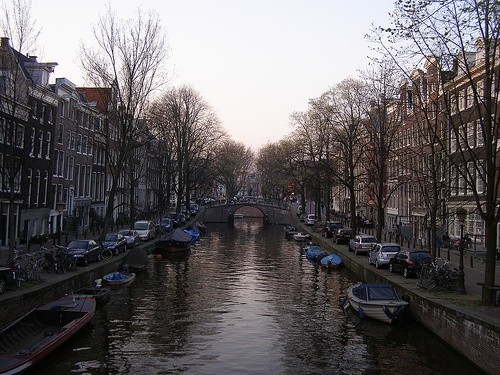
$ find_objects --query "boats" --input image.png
[284,225,344,269]
[102,247,161,285]
[234,212,243,219]
[0,282,112,375]
[342,282,409,324]
[160,221,208,252]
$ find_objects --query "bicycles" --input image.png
[98,242,113,260]
[418,257,460,290]
[6,243,76,288]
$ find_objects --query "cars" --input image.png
[332,228,353,244]
[63,239,101,266]
[102,229,140,256]
[155,204,199,232]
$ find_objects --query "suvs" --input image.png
[304,214,318,225]
[368,242,432,278]
[320,221,344,238]
[349,234,378,255]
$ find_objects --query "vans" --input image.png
[134,220,157,241]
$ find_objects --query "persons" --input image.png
[394,225,400,243]
[356,214,362,226]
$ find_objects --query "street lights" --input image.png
[456,206,467,293]
[357,204,361,235]
